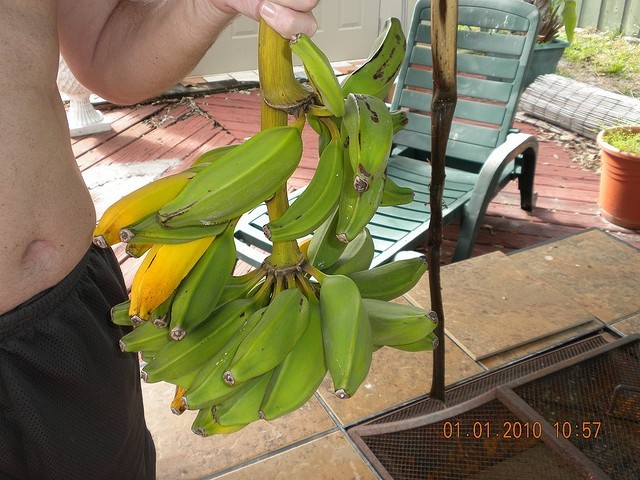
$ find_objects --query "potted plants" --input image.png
[486,0,577,92]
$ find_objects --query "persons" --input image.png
[1,0,321,479]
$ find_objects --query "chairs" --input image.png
[232,0,541,287]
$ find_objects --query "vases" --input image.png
[597,124,640,230]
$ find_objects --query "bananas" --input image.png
[87,17,415,343]
[90,228,440,439]
